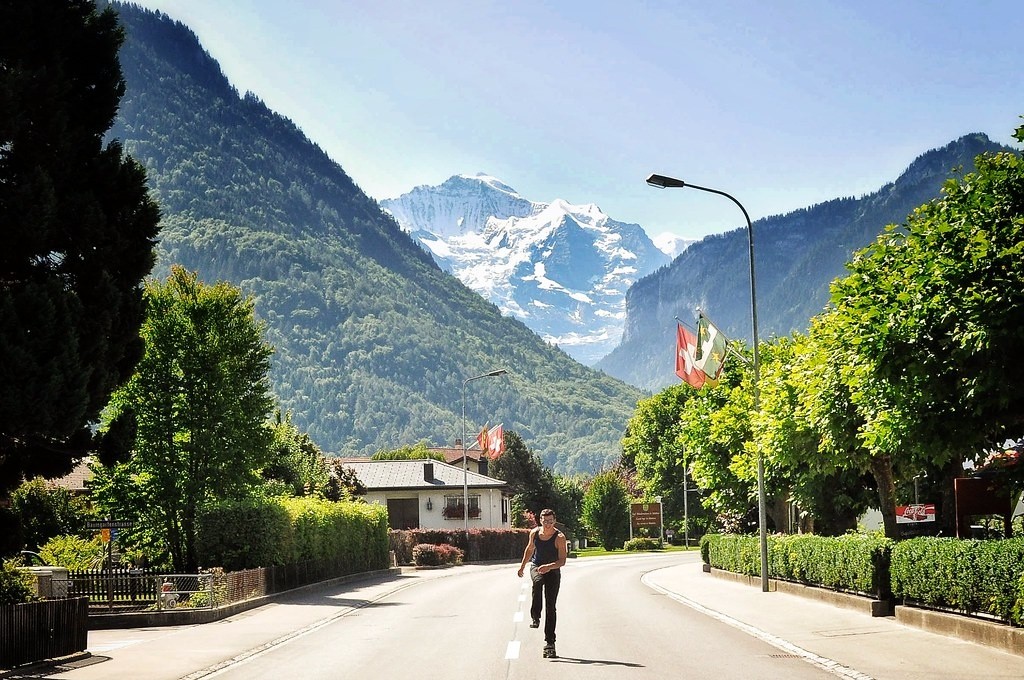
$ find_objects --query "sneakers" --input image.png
[529,617,541,628]
[543,643,555,652]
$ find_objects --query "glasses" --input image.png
[541,519,556,525]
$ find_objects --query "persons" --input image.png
[518,509,566,649]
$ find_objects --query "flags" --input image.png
[674,321,706,389]
[694,311,734,380]
[487,426,504,459]
[477,425,490,451]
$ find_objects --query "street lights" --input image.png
[646,174,769,592]
[955,478,980,538]
[464,369,505,546]
